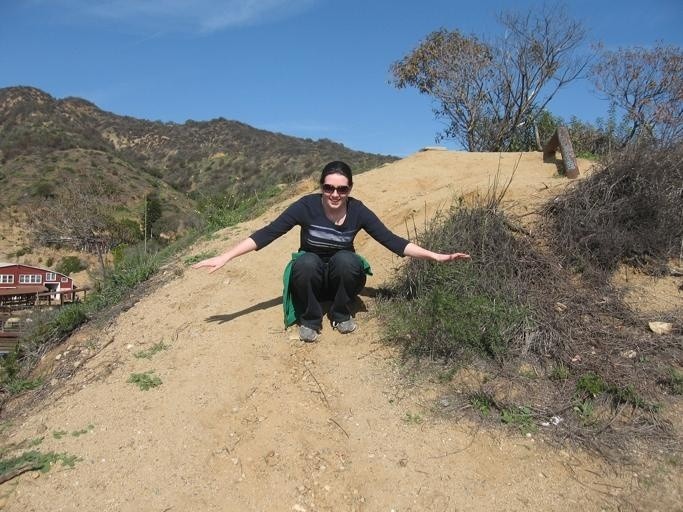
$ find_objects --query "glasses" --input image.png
[320,183,351,195]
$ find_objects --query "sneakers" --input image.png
[299,325,318,342]
[333,313,356,334]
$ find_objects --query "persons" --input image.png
[191,161,470,341]
